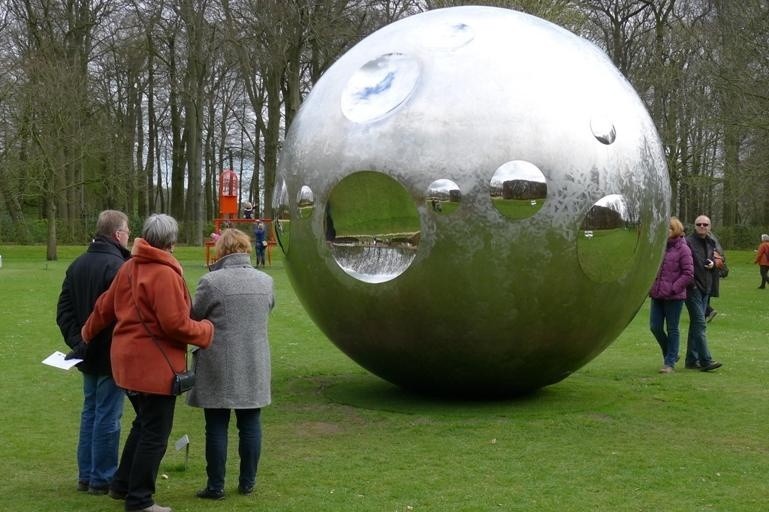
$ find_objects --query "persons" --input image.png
[648,215,695,374]
[253,219,266,269]
[685,215,723,372]
[56,208,134,496]
[208,232,222,242]
[80,212,215,512]
[189,228,275,498]
[753,234,769,289]
[240,201,259,219]
[705,230,729,324]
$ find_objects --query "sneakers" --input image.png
[705,309,717,324]
[758,286,765,290]
[127,504,172,512]
[76,478,89,491]
[685,362,701,369]
[88,485,107,495]
[238,487,254,495]
[196,488,225,499]
[109,488,127,499]
[701,360,722,372]
[673,355,680,363]
[659,366,674,374]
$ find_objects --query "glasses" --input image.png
[119,229,132,236]
[696,223,710,227]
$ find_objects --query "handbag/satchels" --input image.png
[172,371,196,396]
[261,240,268,248]
